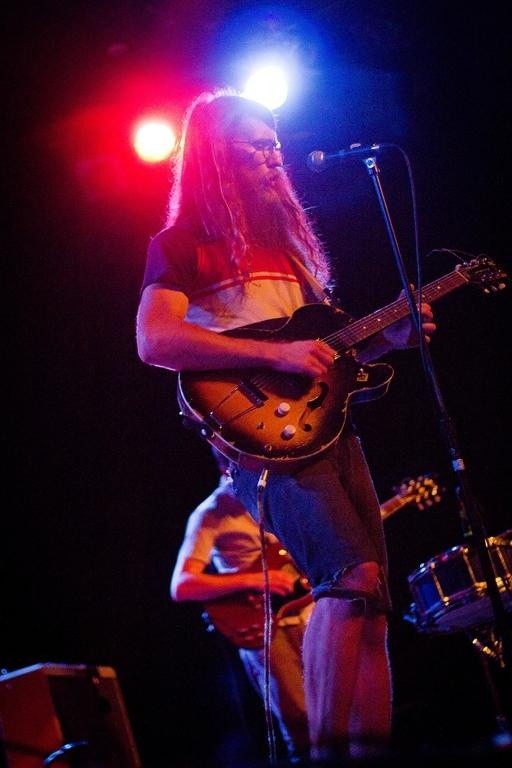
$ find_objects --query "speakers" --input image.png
[5,652,136,759]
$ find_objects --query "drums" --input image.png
[407,531,512,633]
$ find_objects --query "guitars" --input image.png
[200,476,442,650]
[175,255,508,476]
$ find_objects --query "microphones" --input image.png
[305,141,393,174]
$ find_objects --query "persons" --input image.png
[171,445,314,768]
[135,94,435,767]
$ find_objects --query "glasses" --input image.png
[231,138,284,152]
